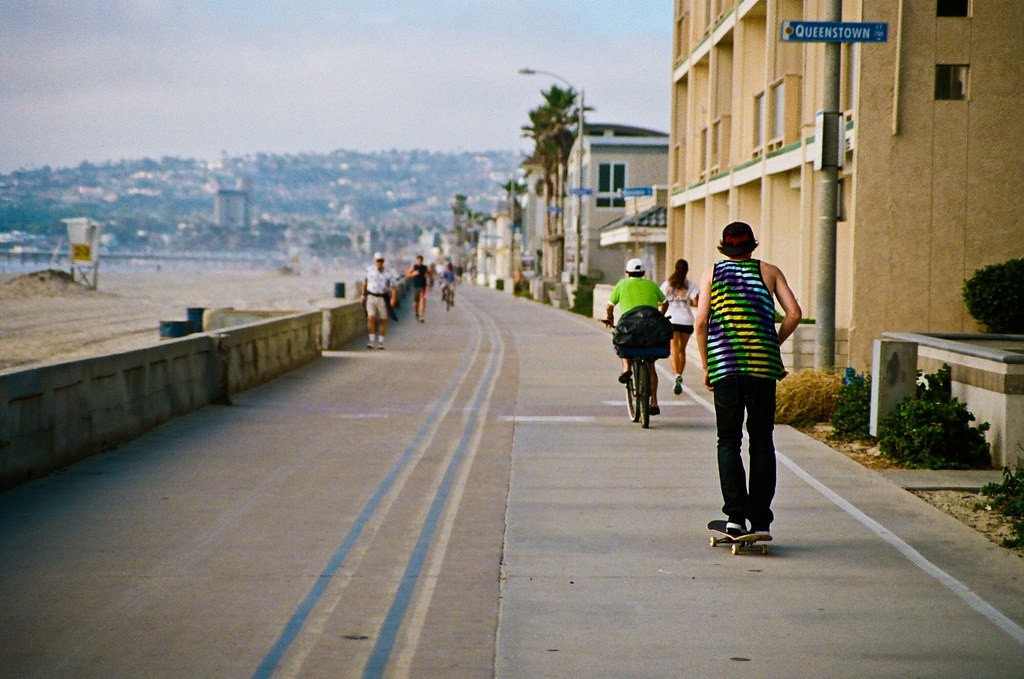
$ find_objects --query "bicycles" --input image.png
[442,278,457,311]
[601,316,672,428]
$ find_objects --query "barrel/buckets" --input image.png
[158,319,196,344]
[188,307,211,333]
[334,281,346,299]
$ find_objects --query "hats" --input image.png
[374,252,384,261]
[626,258,644,272]
[719,222,756,256]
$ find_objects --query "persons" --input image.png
[513,267,524,298]
[694,221,803,536]
[605,258,669,415]
[660,259,700,396]
[404,255,478,322]
[360,253,398,350]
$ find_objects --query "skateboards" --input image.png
[705,519,774,556]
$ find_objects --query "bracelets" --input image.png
[361,293,366,297]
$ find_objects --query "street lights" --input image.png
[518,67,584,293]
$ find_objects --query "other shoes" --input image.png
[442,294,445,300]
[749,525,768,533]
[378,341,384,348]
[650,405,660,415]
[418,317,424,321]
[367,341,374,347]
[726,516,746,534]
[619,369,632,383]
[673,373,682,394]
